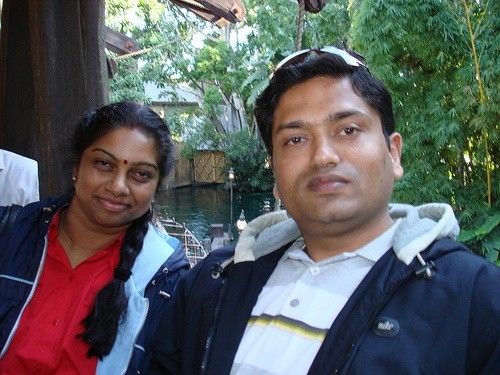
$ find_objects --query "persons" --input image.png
[145,49,500,374]
[0,102,191,375]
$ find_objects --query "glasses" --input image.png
[269,44,372,81]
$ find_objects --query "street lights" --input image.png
[228,167,235,224]
